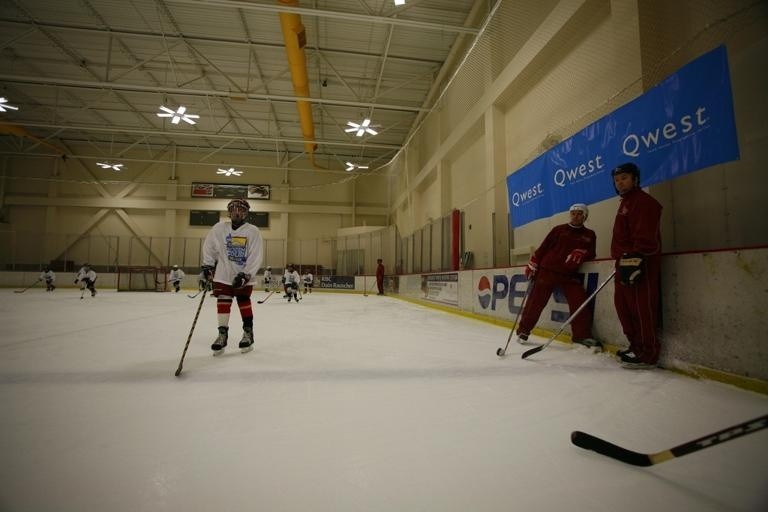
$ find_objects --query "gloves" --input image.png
[565,249,587,264]
[232,272,251,289]
[525,256,539,280]
[198,265,213,291]
[619,251,646,285]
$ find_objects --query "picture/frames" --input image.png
[191,182,270,200]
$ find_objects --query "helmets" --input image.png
[287,264,295,268]
[228,198,250,219]
[569,203,588,222]
[611,162,640,194]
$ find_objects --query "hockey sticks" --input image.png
[186,288,205,298]
[157,278,181,284]
[175,260,218,376]
[497,246,537,355]
[14,280,41,293]
[571,414,768,468]
[522,270,616,359]
[257,281,283,303]
[364,280,376,297]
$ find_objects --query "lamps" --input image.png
[345,156,368,171]
[96,160,124,172]
[0,96,19,113]
[216,167,243,177]
[344,108,378,137]
[156,104,200,125]
[394,0,405,5]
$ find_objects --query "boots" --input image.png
[239,326,253,348]
[211,326,229,350]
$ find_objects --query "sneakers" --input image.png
[516,329,528,340]
[295,297,299,302]
[287,297,291,301]
[617,348,641,363]
[572,336,602,346]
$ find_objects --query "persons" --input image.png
[73,263,97,299]
[199,268,208,291]
[518,203,602,350]
[375,258,384,295]
[264,264,313,301]
[202,197,264,350]
[168,264,185,292]
[610,161,663,365]
[38,266,56,291]
